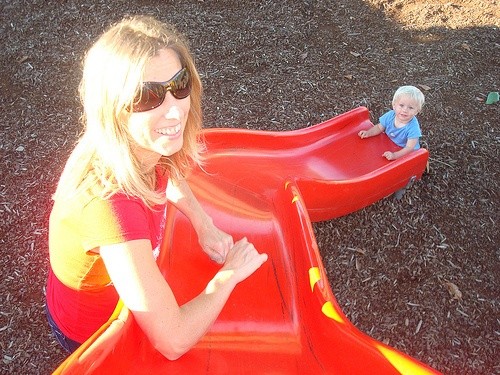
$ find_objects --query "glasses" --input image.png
[125,67,192,113]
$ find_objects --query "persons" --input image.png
[358,85,425,200]
[45,15,269,361]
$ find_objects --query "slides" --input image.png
[51,103,443,375]
